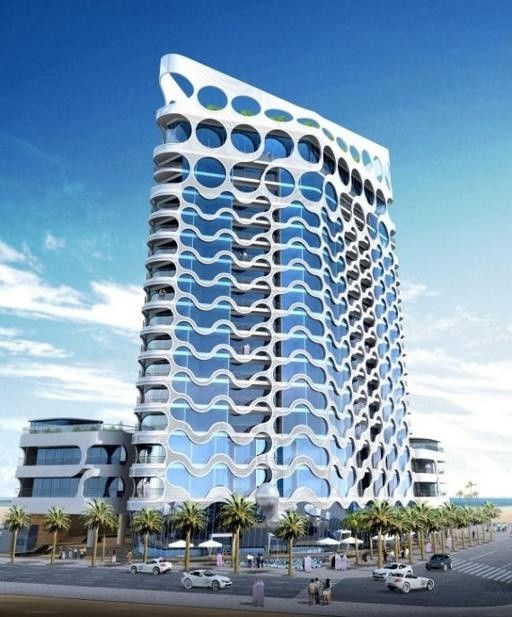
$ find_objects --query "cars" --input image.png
[425,554,453,572]
[373,563,414,581]
[129,558,173,576]
[180,569,233,591]
[385,572,434,594]
